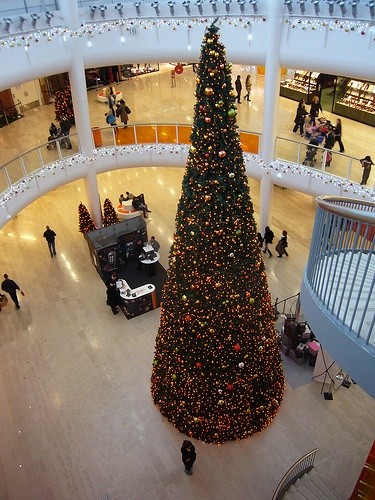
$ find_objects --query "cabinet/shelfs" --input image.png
[331,76,375,127]
[280,70,322,104]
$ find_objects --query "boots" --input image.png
[66,143,72,149]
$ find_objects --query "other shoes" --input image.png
[268,255,272,258]
[112,307,119,315]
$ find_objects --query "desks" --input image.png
[116,279,156,315]
[139,251,160,276]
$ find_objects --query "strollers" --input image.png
[46,128,67,150]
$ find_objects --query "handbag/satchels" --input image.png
[125,106,130,114]
[20,291,24,296]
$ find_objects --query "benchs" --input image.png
[116,202,143,220]
[97,91,122,103]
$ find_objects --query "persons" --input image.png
[360,156,373,185]
[150,236,160,252]
[234,76,241,104]
[119,191,152,218]
[49,83,128,150]
[257,226,289,258]
[244,75,252,101]
[181,440,196,475]
[43,226,56,259]
[292,96,345,166]
[105,273,121,315]
[0,274,23,311]
